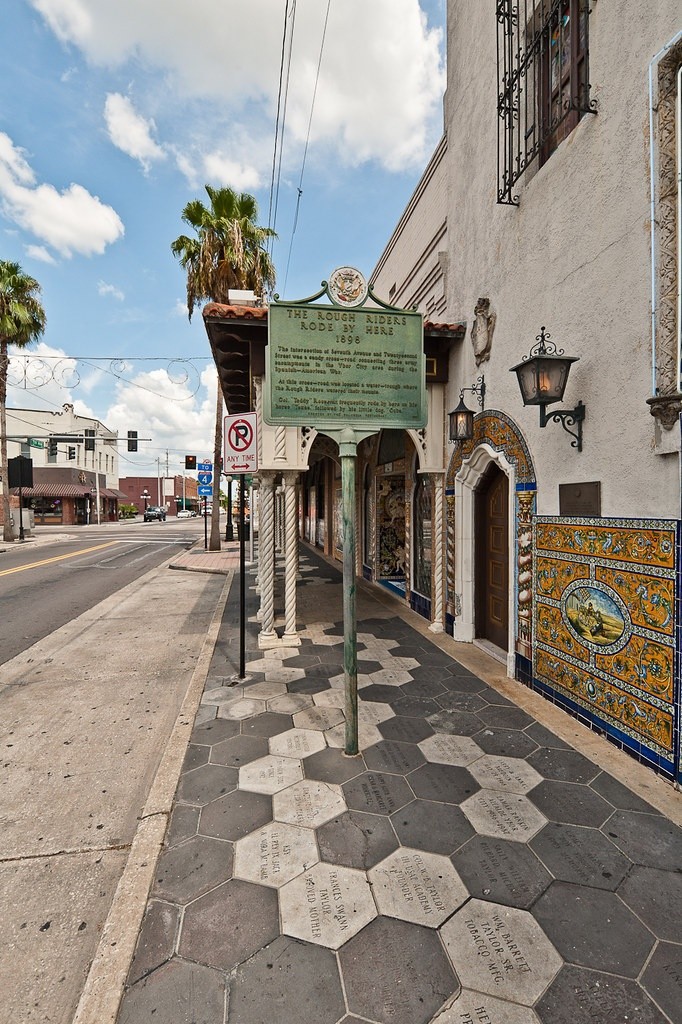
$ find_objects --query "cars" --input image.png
[191,511,196,517]
[177,509,192,518]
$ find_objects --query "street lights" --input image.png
[224,476,234,541]
[140,489,151,522]
[173,495,181,517]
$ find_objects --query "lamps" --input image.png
[508,326,585,452]
[448,375,486,451]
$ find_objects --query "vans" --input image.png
[200,505,213,517]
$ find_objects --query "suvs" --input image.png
[144,506,167,522]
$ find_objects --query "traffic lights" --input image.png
[220,458,223,470]
[49,441,57,456]
[185,455,197,470]
[68,446,76,460]
